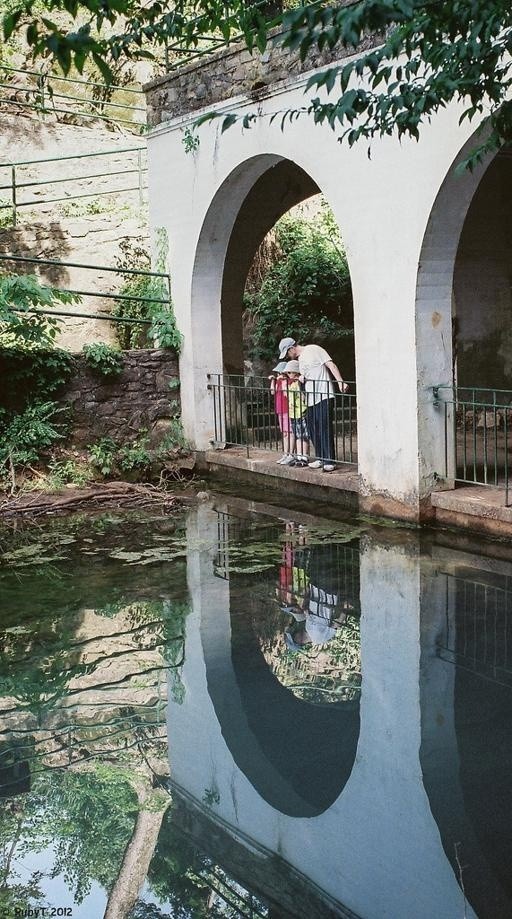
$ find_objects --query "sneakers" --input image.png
[295,459,310,466]
[288,458,299,466]
[323,464,337,471]
[308,459,323,469]
[276,455,289,463]
[280,457,294,465]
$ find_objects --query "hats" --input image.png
[278,337,296,361]
[272,362,287,373]
[282,360,300,374]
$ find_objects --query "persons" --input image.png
[279,337,350,472]
[278,360,310,466]
[268,362,295,465]
[275,522,354,651]
